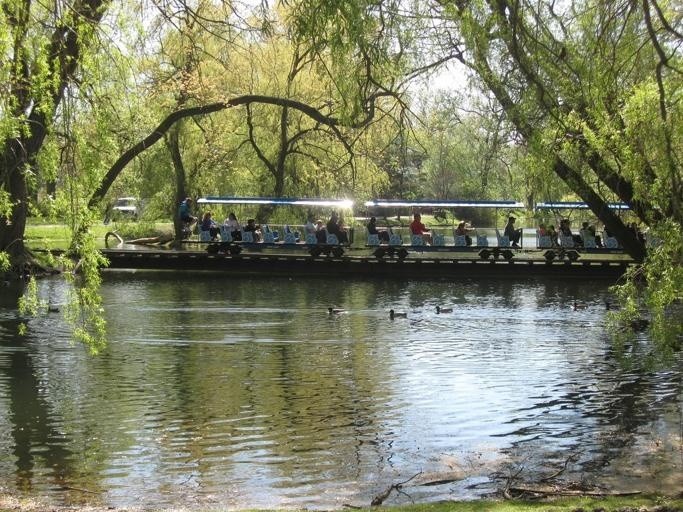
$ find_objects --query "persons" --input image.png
[203,212,261,242]
[455,221,472,247]
[505,217,521,247]
[304,214,348,243]
[367,216,383,244]
[540,218,642,248]
[178,198,198,235]
[410,214,432,244]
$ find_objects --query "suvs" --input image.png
[109,196,139,223]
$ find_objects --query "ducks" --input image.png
[48,307,59,312]
[328,308,344,312]
[436,306,453,314]
[574,303,587,307]
[389,310,407,320]
[606,303,618,310]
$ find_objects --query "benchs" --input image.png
[453,230,473,246]
[602,231,618,248]
[431,228,445,246]
[198,224,212,241]
[326,228,340,245]
[221,224,233,240]
[284,223,301,244]
[559,229,575,247]
[495,229,513,247]
[581,229,596,248]
[409,226,431,246]
[366,227,383,245]
[387,226,404,245]
[304,222,317,243]
[475,228,488,246]
[241,225,261,242]
[535,230,552,248]
[264,225,279,243]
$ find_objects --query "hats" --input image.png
[508,216,516,221]
